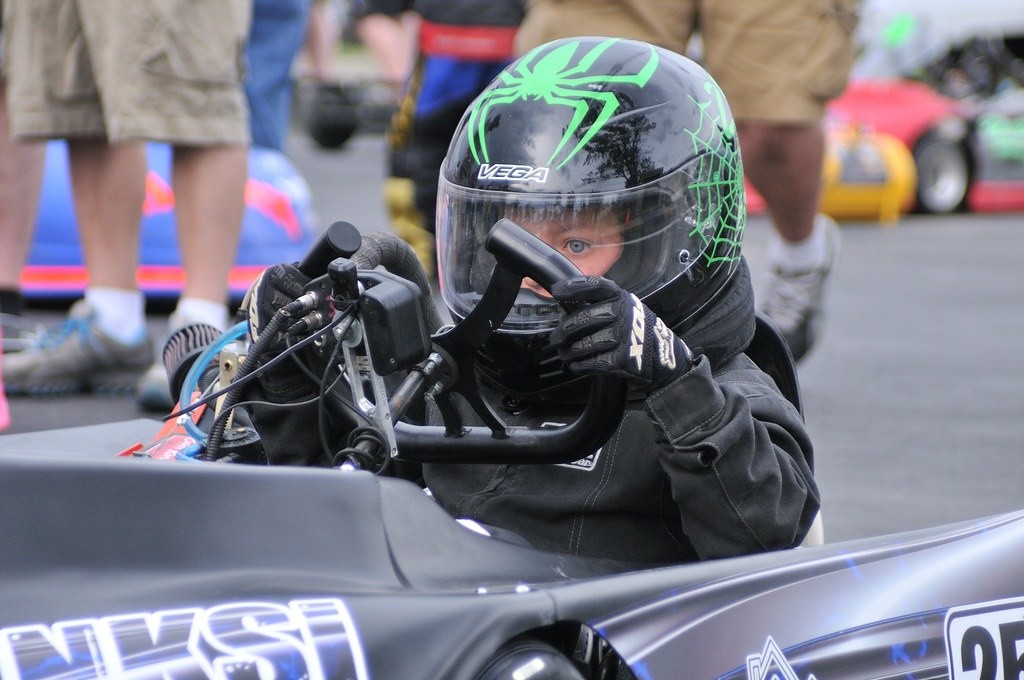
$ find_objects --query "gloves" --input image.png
[246,261,313,361]
[554,276,694,396]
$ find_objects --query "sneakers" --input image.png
[135,361,175,411]
[3,300,156,397]
[764,217,836,360]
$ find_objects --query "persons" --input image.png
[197,35,824,562]
[0,0,866,415]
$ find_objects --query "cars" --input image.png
[803,28,1024,227]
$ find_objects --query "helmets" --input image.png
[437,37,745,398]
